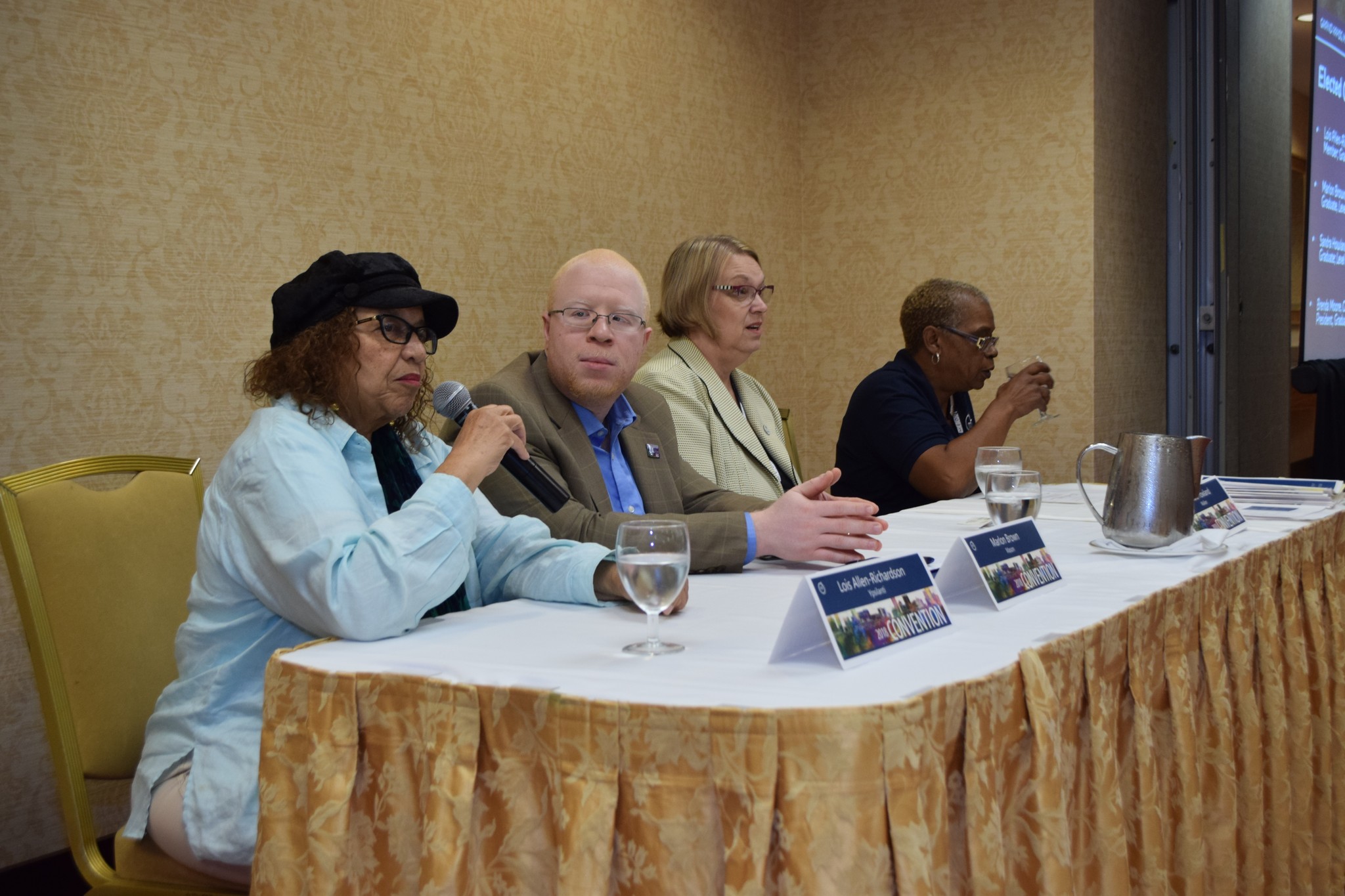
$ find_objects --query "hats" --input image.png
[270,251,459,348]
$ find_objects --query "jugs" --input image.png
[1077,431,1213,548]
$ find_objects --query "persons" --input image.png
[827,278,1054,515]
[435,249,892,573]
[623,238,805,503]
[122,249,688,887]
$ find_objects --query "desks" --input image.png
[246,477,1345,896]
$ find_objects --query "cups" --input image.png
[985,471,1041,525]
[974,447,1023,497]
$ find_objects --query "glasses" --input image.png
[549,308,647,335]
[710,285,774,308]
[934,324,999,352]
[352,314,438,354]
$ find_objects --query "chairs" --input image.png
[0,454,247,896]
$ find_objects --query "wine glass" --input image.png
[1005,355,1061,426]
[614,519,691,655]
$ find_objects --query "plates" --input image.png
[1090,539,1228,556]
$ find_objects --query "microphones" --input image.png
[433,380,571,515]
